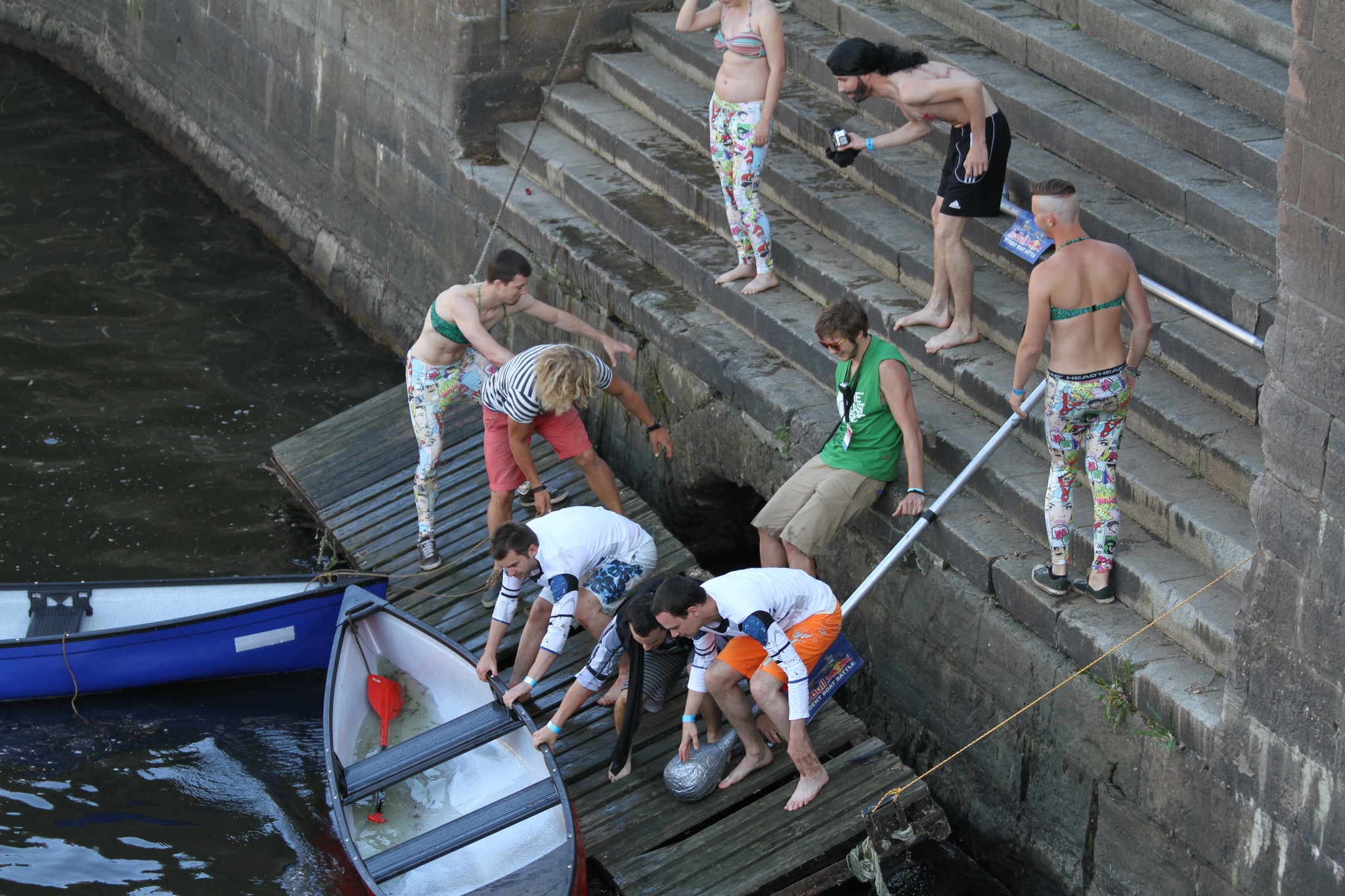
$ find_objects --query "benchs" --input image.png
[361,777,561,884]
[339,701,526,805]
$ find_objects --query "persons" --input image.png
[532,578,786,784]
[1009,181,1155,604]
[751,300,926,582]
[824,35,1013,355]
[404,248,638,571]
[482,342,673,606]
[675,0,786,295]
[475,505,658,707]
[651,566,843,812]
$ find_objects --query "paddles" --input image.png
[367,673,404,825]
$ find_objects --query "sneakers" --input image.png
[1032,563,1068,596]
[634,521,654,542]
[416,535,441,571]
[482,570,504,608]
[517,482,569,506]
[1071,573,1115,604]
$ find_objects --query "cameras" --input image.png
[826,126,860,168]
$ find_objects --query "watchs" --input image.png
[645,418,662,432]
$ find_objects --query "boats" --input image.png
[322,585,587,896]
[1,573,390,704]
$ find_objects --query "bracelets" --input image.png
[547,720,562,734]
[531,483,546,493]
[1125,363,1142,380]
[907,488,925,496]
[523,675,538,688]
[866,138,874,153]
[1011,381,1024,395]
[682,715,696,723]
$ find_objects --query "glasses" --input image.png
[818,337,848,351]
